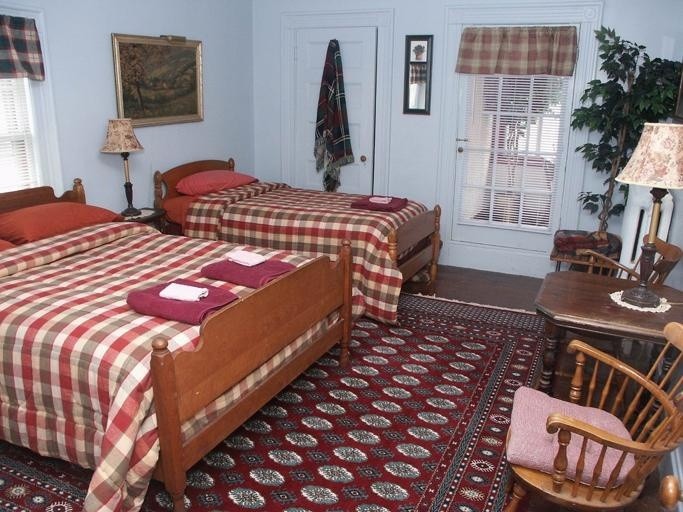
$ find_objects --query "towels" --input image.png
[350,194,410,213]
[369,196,393,204]
[201,254,299,289]
[225,249,269,268]
[158,280,212,305]
[124,277,241,326]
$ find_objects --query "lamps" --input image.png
[100,119,146,218]
[611,120,682,308]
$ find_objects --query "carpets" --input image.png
[0,284,558,511]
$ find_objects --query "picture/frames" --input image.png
[108,33,208,129]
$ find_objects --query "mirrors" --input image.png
[401,32,435,115]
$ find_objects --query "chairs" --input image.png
[503,321,683,512]
[570,236,682,286]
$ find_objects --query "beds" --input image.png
[151,156,447,325]
[0,178,356,512]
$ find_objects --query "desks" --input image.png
[546,229,622,273]
[527,269,683,440]
[124,206,167,233]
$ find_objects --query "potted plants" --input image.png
[412,43,427,63]
[566,24,681,276]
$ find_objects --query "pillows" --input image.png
[0,200,119,246]
[0,239,17,253]
[173,169,261,196]
